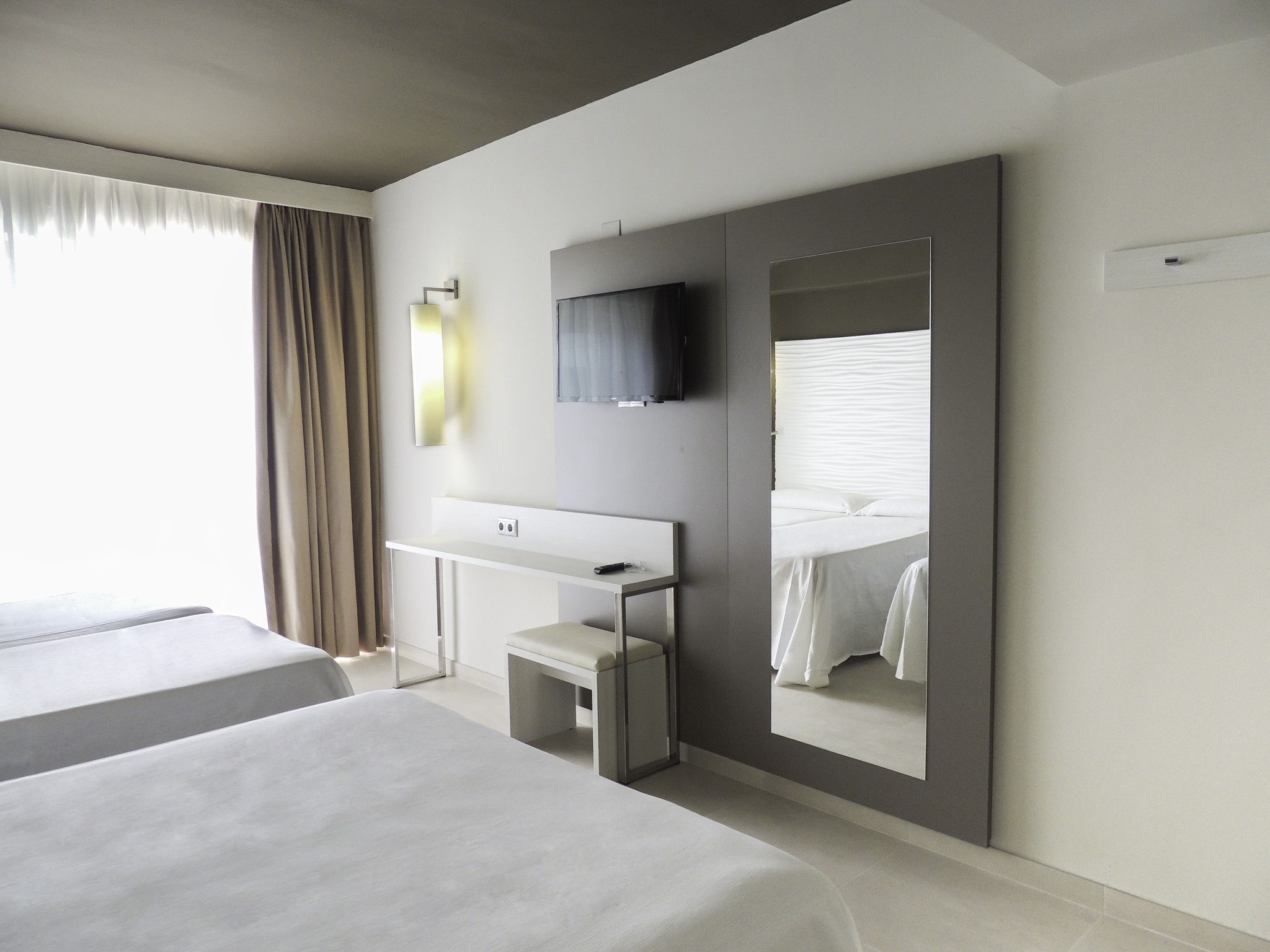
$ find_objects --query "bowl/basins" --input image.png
[624,560,646,572]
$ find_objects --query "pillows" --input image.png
[771,487,878,516]
[852,493,930,518]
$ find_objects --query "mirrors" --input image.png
[769,236,933,779]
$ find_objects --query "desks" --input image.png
[386,497,680,785]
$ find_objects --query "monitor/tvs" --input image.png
[557,281,686,409]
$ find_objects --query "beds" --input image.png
[0,590,356,782]
[770,486,855,528]
[878,558,927,684]
[771,492,930,690]
[0,688,864,952]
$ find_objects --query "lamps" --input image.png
[410,279,458,446]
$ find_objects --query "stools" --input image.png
[503,621,667,782]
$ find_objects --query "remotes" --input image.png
[594,562,632,575]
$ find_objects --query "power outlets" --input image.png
[498,517,518,537]
